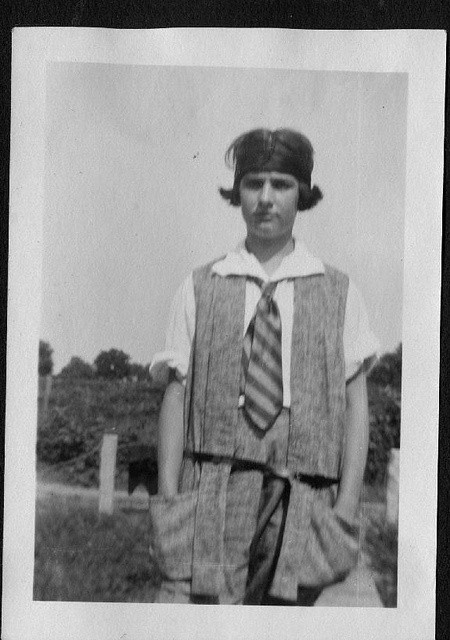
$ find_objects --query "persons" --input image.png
[150,127,386,606]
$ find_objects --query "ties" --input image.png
[240,275,283,432]
